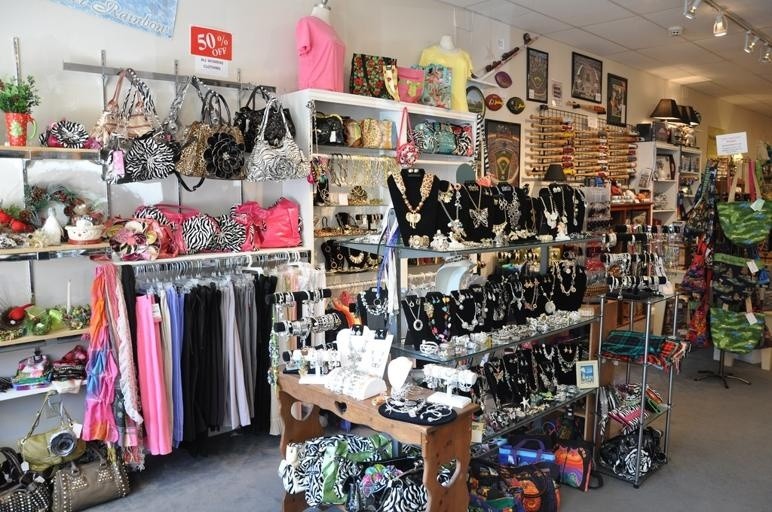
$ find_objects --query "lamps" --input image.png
[648,98,701,147]
[683,0,772,65]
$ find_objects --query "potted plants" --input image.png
[0,76,39,148]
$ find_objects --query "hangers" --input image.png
[126,246,313,286]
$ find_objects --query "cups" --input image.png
[5,112,38,148]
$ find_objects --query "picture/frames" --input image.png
[571,50,604,104]
[526,47,549,104]
[607,72,628,128]
[465,85,487,125]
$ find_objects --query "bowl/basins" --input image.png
[65,224,105,244]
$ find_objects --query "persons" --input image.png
[294,1,346,92]
[419,35,472,114]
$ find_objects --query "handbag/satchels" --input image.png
[20,389,87,471]
[162,74,225,143]
[397,144,418,164]
[107,217,162,259]
[379,468,452,512]
[133,205,221,253]
[468,462,524,512]
[363,120,398,150]
[499,445,556,470]
[684,158,710,236]
[717,163,772,248]
[713,254,764,303]
[350,53,397,99]
[176,92,245,180]
[102,76,180,183]
[231,198,303,248]
[54,446,131,511]
[490,462,561,512]
[709,294,765,355]
[0,448,52,511]
[679,240,707,292]
[396,67,425,102]
[246,97,311,181]
[341,117,361,148]
[213,216,257,252]
[303,433,392,507]
[312,114,344,144]
[342,455,422,511]
[415,123,456,153]
[234,85,296,154]
[451,125,472,155]
[554,444,591,491]
[686,293,708,347]
[281,433,352,493]
[38,121,92,148]
[414,64,452,110]
[92,70,156,144]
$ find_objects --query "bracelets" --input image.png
[273,288,343,373]
[600,223,666,300]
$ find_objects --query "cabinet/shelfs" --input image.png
[0,146,114,403]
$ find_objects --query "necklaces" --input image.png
[313,152,401,275]
[389,170,435,230]
[402,183,585,423]
[359,290,388,317]
